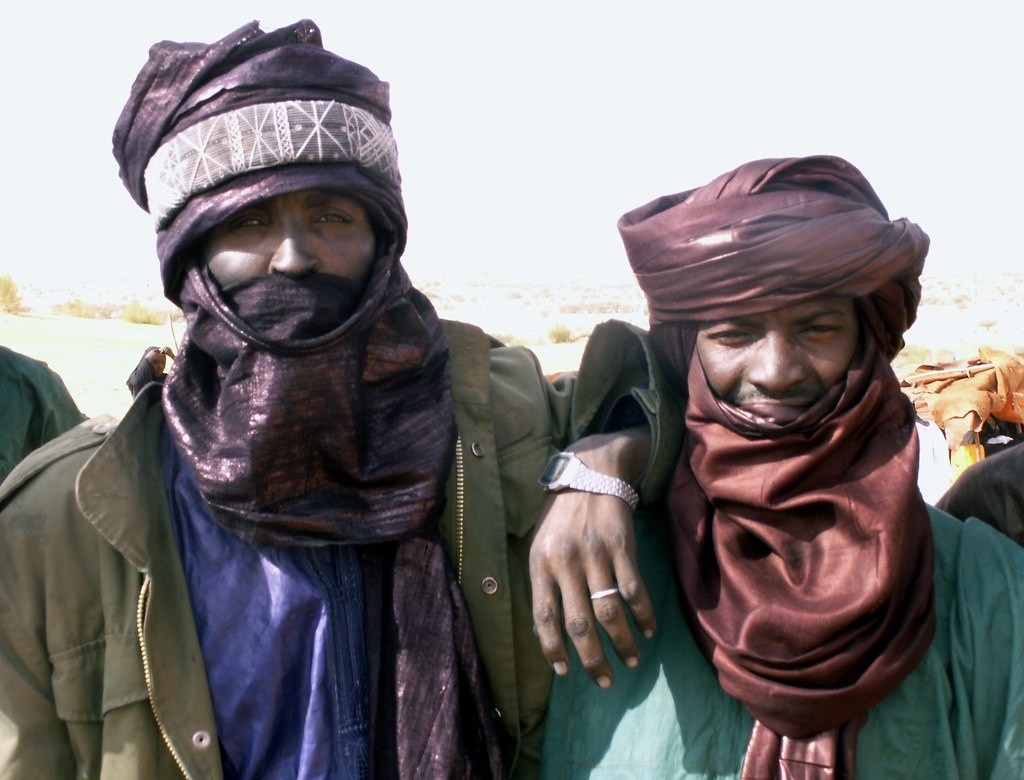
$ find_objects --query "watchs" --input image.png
[538,451,639,509]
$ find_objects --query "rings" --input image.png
[590,588,618,600]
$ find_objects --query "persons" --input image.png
[0,344,88,486]
[0,20,684,779]
[933,440,1024,546]
[541,154,1024,780]
[126,346,177,400]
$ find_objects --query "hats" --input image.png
[620,151,934,351]
[96,28,414,254]
